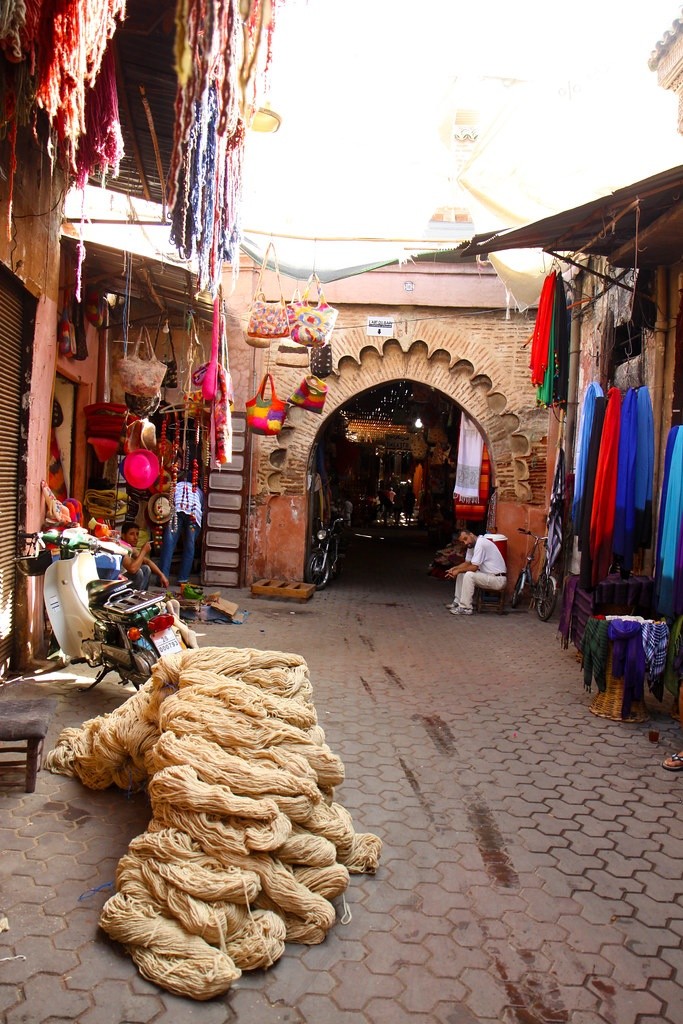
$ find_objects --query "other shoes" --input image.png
[155,579,165,587]
[177,580,190,586]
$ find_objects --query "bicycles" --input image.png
[510,527,559,620]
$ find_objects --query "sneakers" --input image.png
[446,602,472,614]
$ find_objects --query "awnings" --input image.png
[454,163,683,257]
[61,232,216,330]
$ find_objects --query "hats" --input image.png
[124,418,156,454]
[124,449,159,489]
[88,436,119,462]
[149,467,173,494]
[125,390,161,419]
[148,493,174,524]
[156,439,185,479]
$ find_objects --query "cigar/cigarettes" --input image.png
[149,541,154,543]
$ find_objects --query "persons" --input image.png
[157,472,204,587]
[341,485,415,529]
[444,529,507,615]
[118,522,169,592]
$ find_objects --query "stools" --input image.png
[0,698,58,794]
[476,588,505,615]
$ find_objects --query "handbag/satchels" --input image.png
[286,272,339,347]
[201,362,217,400]
[310,344,332,379]
[60,287,104,359]
[276,345,308,367]
[119,354,178,398]
[287,375,328,415]
[247,243,290,338]
[246,374,289,434]
[243,330,271,348]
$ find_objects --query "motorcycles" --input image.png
[10,530,187,693]
[307,515,349,591]
